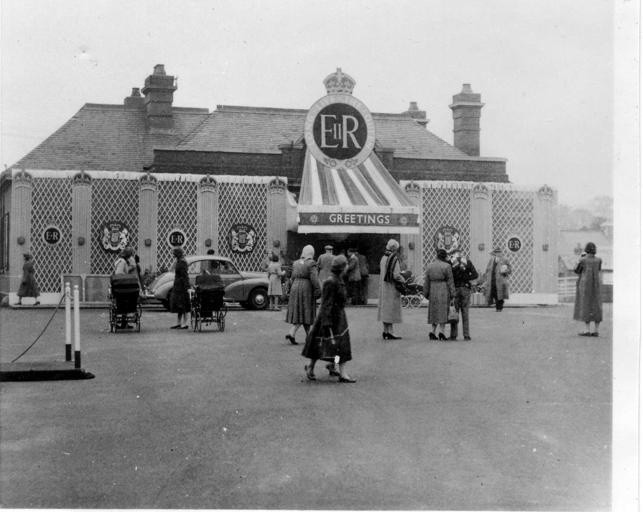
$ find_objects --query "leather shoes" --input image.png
[305,364,316,380]
[177,326,188,329]
[170,325,181,329]
[464,336,471,341]
[592,332,598,336]
[451,336,456,340]
[579,332,591,336]
[338,375,356,383]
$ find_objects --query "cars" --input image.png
[148,252,271,307]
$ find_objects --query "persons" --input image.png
[16,252,43,306]
[112,247,140,328]
[324,358,341,377]
[573,243,603,338]
[301,255,358,383]
[170,248,191,327]
[448,249,479,339]
[210,261,233,274]
[484,246,513,313]
[344,248,369,304]
[424,248,455,340]
[377,239,412,338]
[268,255,285,310]
[318,246,336,281]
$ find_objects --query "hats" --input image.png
[493,247,501,252]
[325,245,333,250]
[331,254,346,266]
[386,239,399,250]
[585,242,596,253]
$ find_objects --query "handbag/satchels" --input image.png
[448,305,459,323]
[396,280,407,296]
[315,335,343,360]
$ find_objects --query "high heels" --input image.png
[429,333,438,340]
[383,332,387,339]
[439,333,447,340]
[286,334,298,344]
[387,332,402,339]
